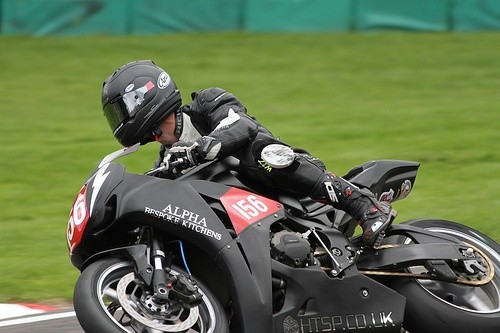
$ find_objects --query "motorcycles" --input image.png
[68,143,499,333]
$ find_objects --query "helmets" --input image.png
[102,60,182,146]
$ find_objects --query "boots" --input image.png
[285,153,398,244]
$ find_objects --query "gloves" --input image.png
[161,142,205,176]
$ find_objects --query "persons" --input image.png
[101,59,397,245]
